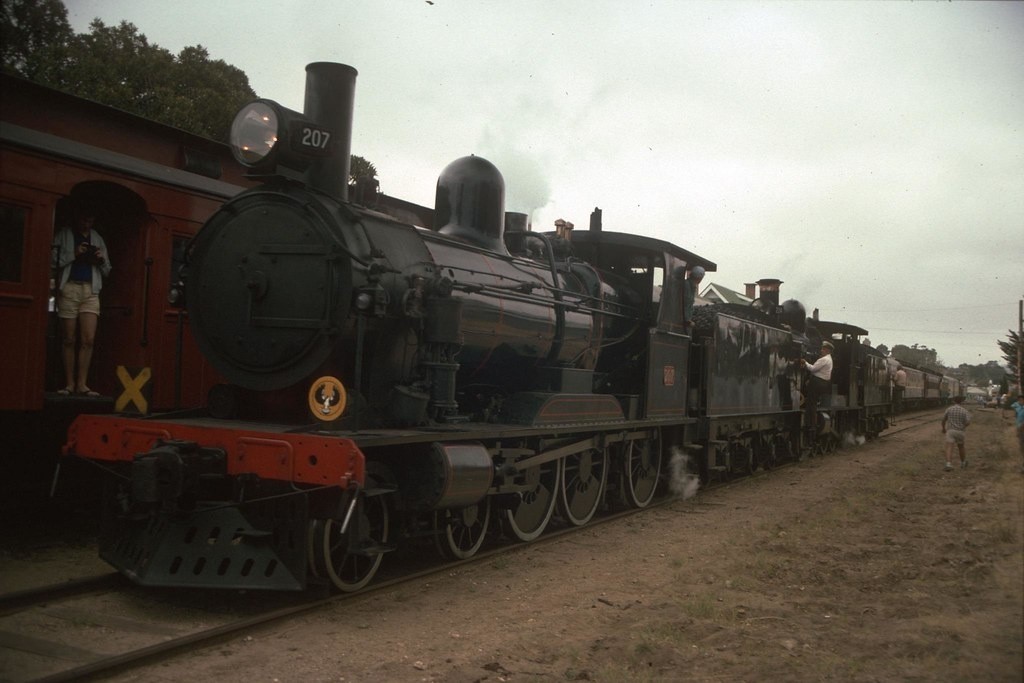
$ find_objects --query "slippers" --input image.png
[73,389,103,399]
[57,388,76,395]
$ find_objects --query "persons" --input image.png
[941,396,971,471]
[946,389,951,396]
[1010,396,1024,456]
[769,350,795,410]
[801,341,833,427]
[893,365,907,411]
[999,392,1010,421]
[683,266,705,336]
[55,206,113,398]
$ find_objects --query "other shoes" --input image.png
[944,465,954,471]
[961,461,968,468]
[808,426,818,431]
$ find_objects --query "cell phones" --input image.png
[84,245,97,252]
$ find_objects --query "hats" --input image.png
[820,341,835,351]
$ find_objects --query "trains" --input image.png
[0,68,971,417]
[44,59,899,595]
